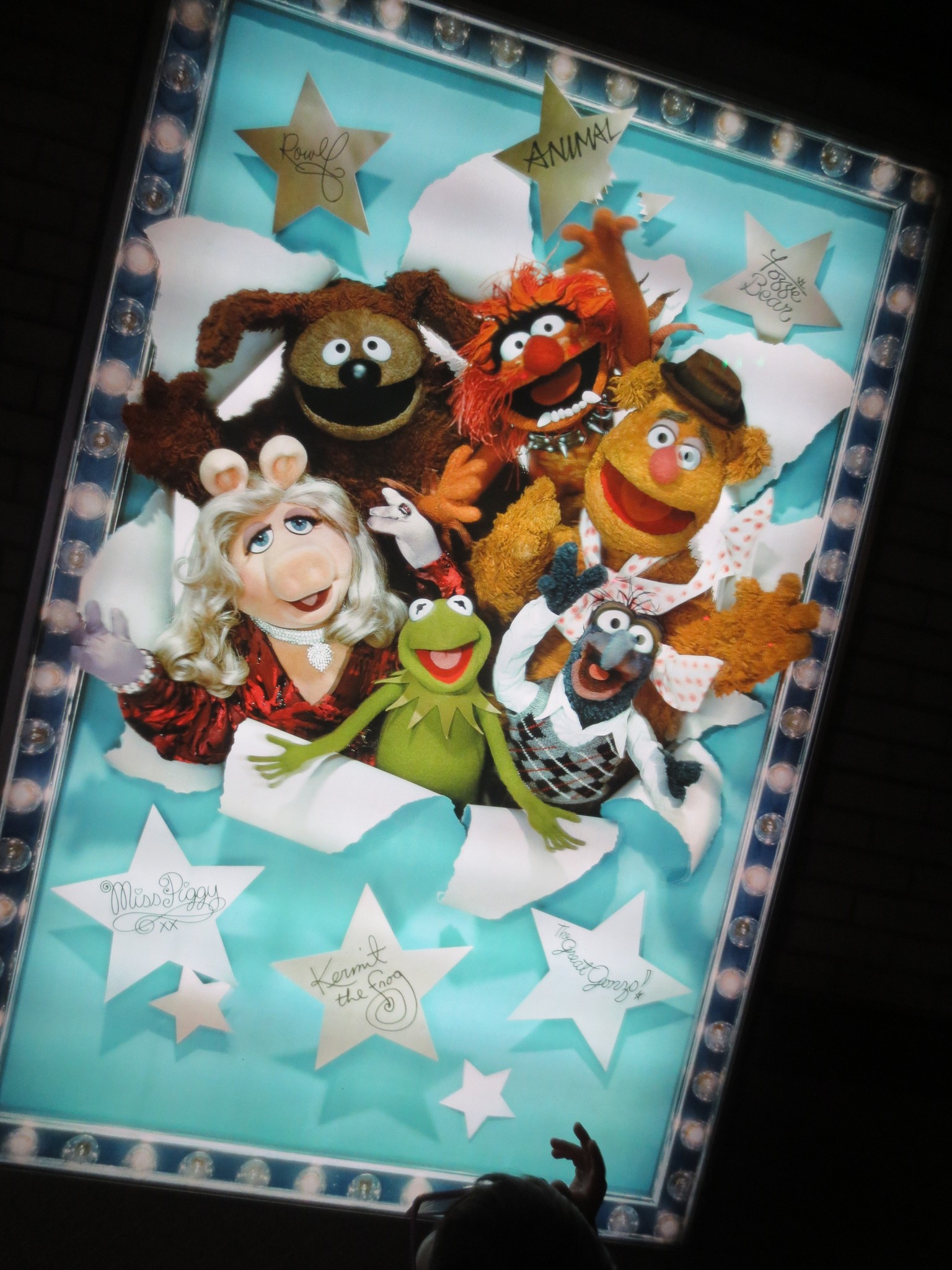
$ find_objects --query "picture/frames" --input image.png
[0,0,952,1257]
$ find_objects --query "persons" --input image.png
[402,1122,617,1270]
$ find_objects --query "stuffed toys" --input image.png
[71,209,819,851]
